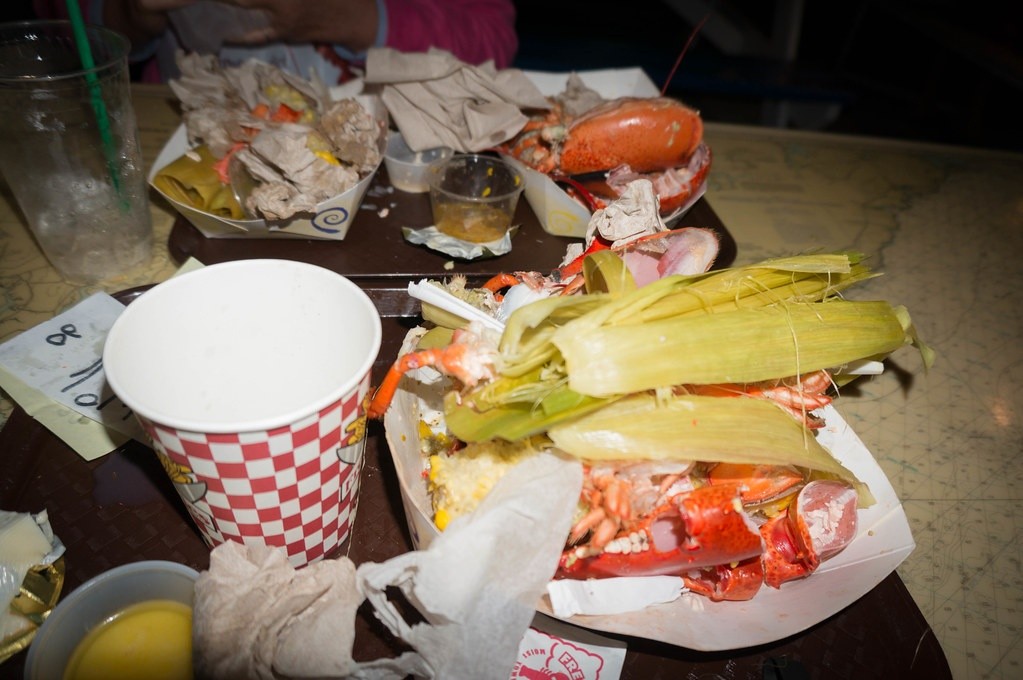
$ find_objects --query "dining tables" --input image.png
[1,61,1022,678]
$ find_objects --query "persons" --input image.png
[38,0,520,94]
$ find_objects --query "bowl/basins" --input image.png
[422,154,528,242]
[383,133,454,193]
[26,559,201,680]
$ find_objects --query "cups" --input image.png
[1,20,156,288]
[103,256,378,569]
